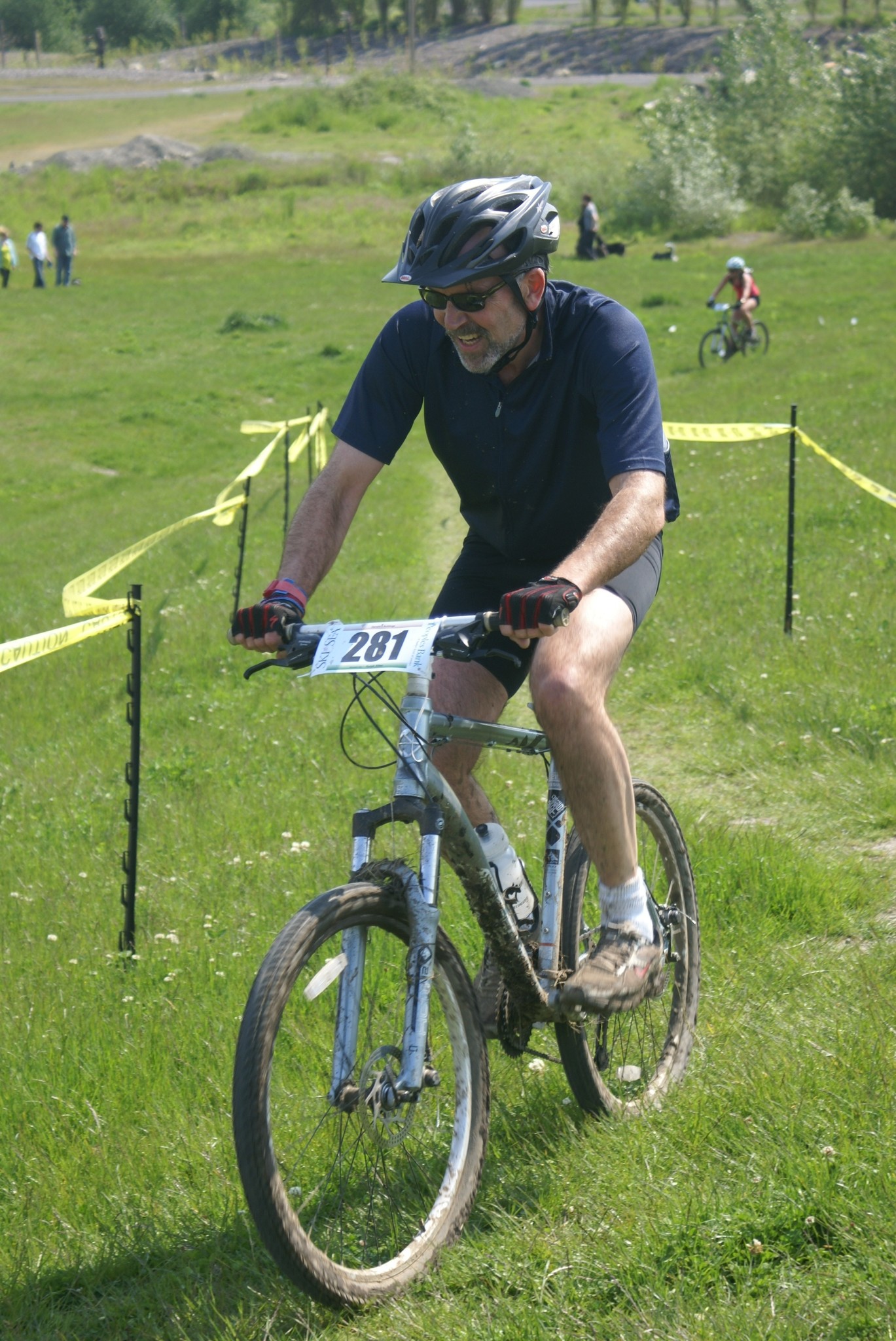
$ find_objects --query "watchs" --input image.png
[262,580,307,606]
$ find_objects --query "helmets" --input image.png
[381,174,564,287]
[726,256,746,269]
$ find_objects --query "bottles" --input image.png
[473,822,534,919]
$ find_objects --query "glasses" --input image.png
[417,264,548,313]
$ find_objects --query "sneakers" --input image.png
[561,925,665,1016]
[472,906,544,1039]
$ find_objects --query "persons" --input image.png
[51,215,75,286]
[26,222,51,287]
[707,257,760,350]
[576,194,607,260]
[0,226,16,288]
[229,175,679,1036]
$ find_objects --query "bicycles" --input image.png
[233,607,701,1308]
[698,301,770,369]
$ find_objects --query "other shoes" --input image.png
[745,329,757,339]
